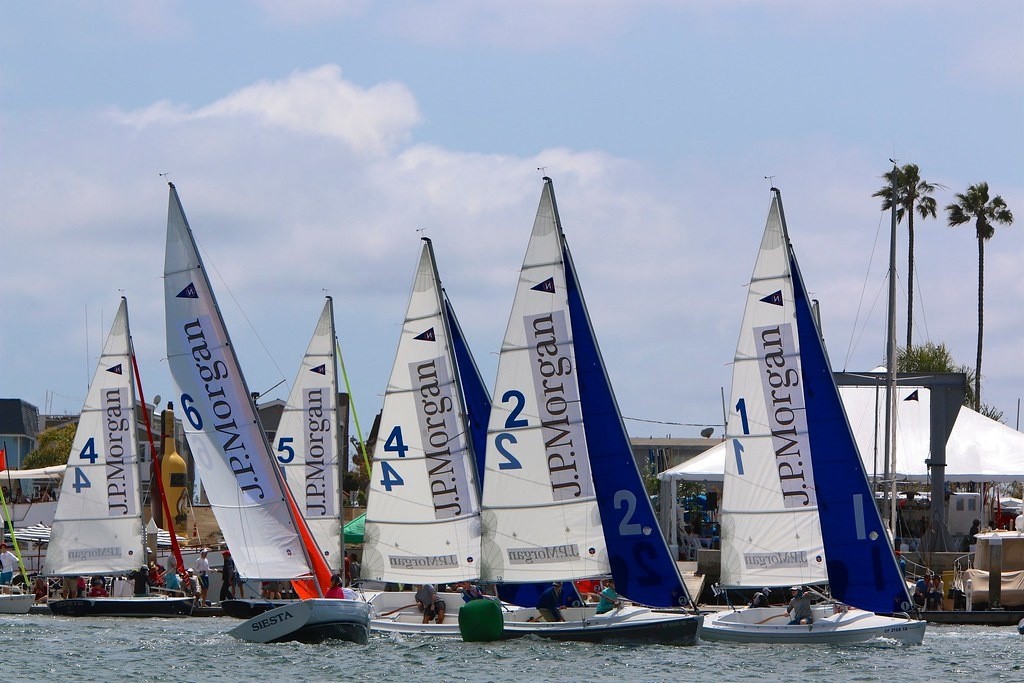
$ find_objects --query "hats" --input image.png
[150,564,159,572]
[331,574,340,581]
[789,585,802,591]
[973,519,981,523]
[200,549,208,555]
[350,553,357,557]
[187,568,194,574]
[0,543,7,549]
[221,551,230,556]
[146,547,153,554]
[95,579,103,585]
[895,551,900,555]
[763,586,772,593]
[553,582,562,587]
[141,565,150,571]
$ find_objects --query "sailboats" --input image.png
[476,169,704,646]
[223,236,493,620]
[0,483,37,615]
[699,175,933,651]
[40,295,199,615]
[163,178,375,645]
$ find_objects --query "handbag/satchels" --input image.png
[77,575,85,588]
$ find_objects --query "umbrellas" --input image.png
[337,511,367,545]
[4,521,51,578]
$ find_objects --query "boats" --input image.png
[918,611,1024,625]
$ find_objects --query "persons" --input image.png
[343,549,362,592]
[90,575,107,598]
[783,585,822,625]
[595,579,621,615]
[748,587,772,608]
[324,574,345,599]
[128,560,166,597]
[178,548,244,607]
[969,519,981,545]
[62,575,82,600]
[0,543,19,595]
[162,545,188,597]
[460,581,485,603]
[535,581,568,622]
[0,485,56,505]
[913,573,946,611]
[413,584,447,624]
[893,550,906,585]
